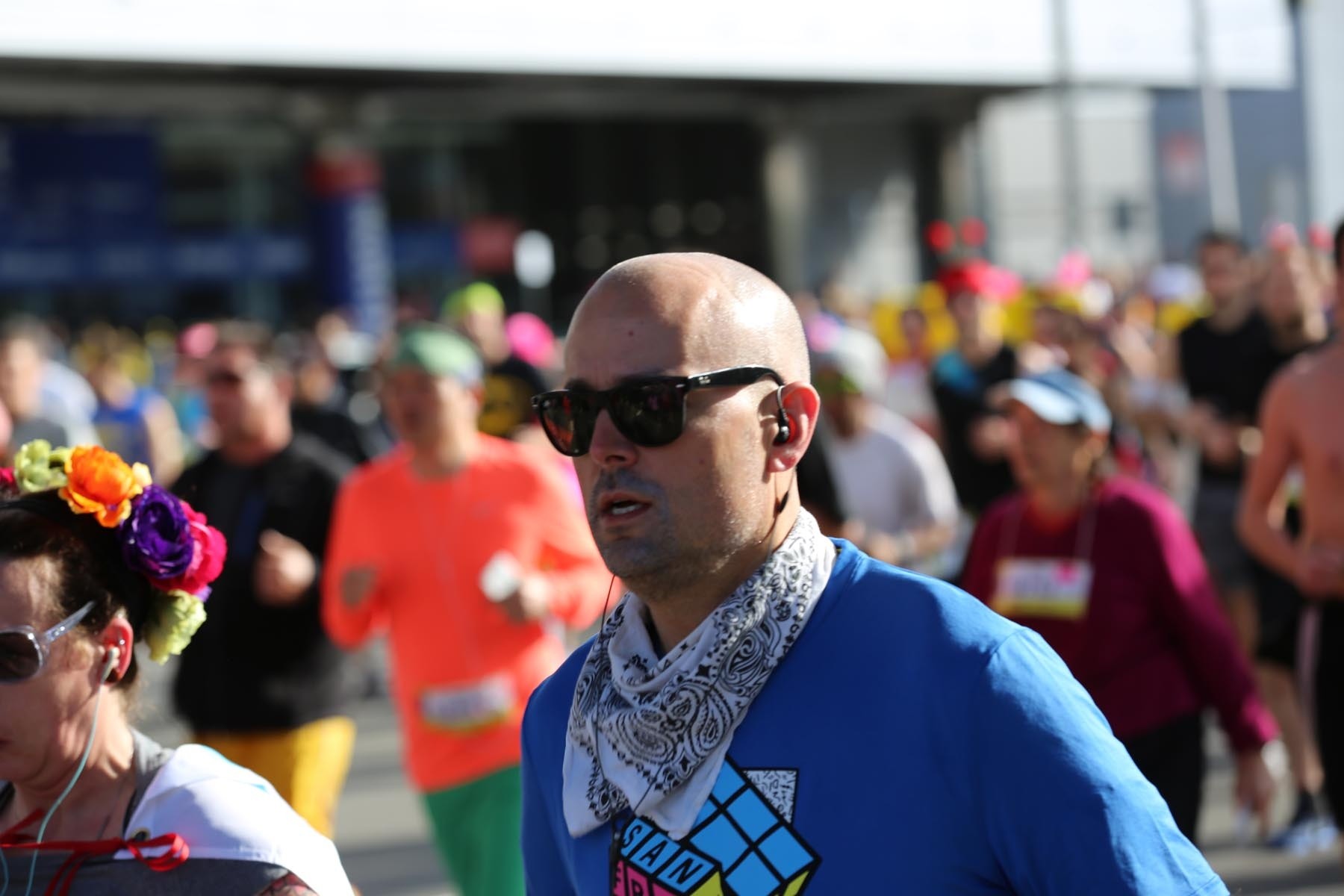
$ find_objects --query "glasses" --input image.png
[530,367,785,458]
[0,598,95,684]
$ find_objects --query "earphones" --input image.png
[96,647,120,682]
[772,384,792,446]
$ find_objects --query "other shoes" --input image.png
[1280,791,1338,847]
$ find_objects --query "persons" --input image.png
[959,363,1298,850]
[1,441,356,896]
[163,349,357,841]
[520,254,1236,896]
[0,223,1344,860]
[321,320,628,896]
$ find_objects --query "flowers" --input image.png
[0,438,228,662]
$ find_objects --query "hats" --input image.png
[986,363,1112,434]
[439,283,508,319]
[381,327,482,385]
[809,327,886,401]
